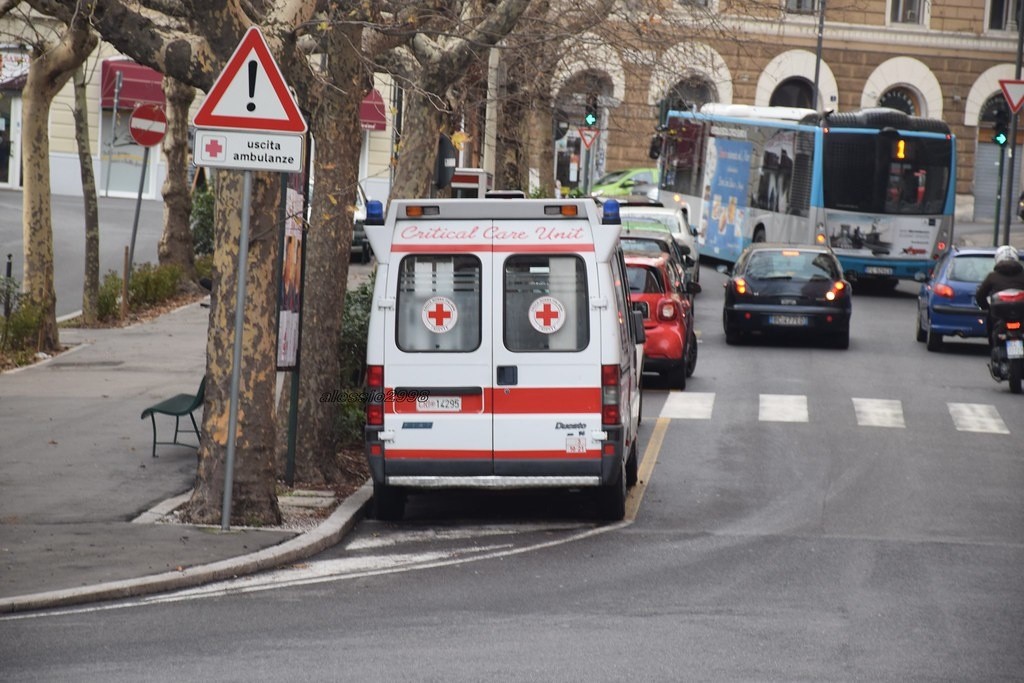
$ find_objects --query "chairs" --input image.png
[139,374,209,458]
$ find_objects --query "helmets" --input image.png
[996,244,1017,264]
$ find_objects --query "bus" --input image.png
[648,102,957,294]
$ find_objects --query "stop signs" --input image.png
[130,103,168,147]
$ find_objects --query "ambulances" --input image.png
[363,196,645,525]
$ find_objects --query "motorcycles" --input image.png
[987,288,1024,395]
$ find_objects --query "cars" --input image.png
[619,206,702,282]
[621,250,702,391]
[620,228,696,316]
[351,184,374,262]
[915,245,1024,352]
[568,167,661,196]
[620,218,691,256]
[716,242,858,349]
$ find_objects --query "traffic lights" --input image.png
[585,92,597,126]
[993,110,1010,146]
